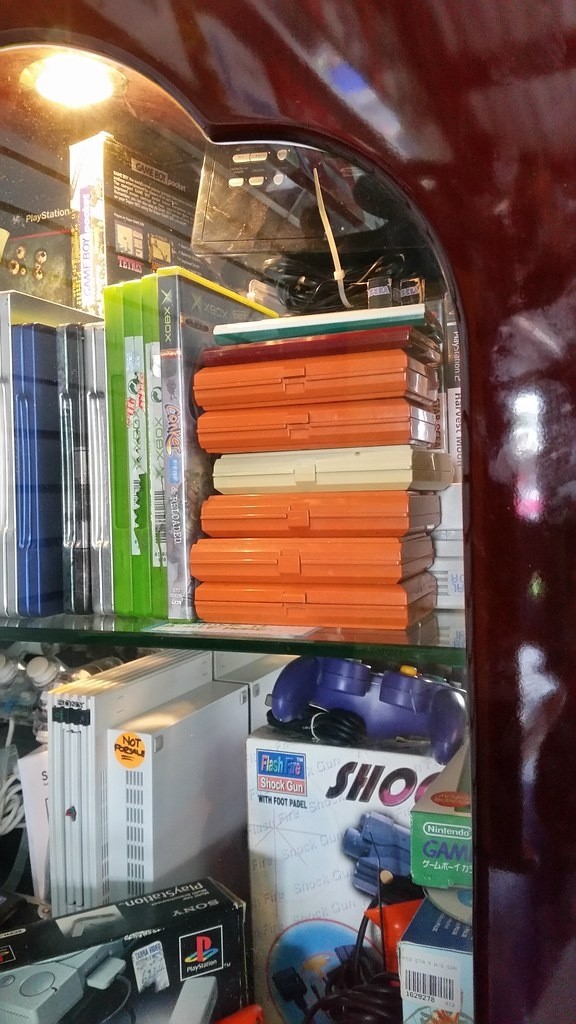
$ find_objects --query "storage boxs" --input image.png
[69,130,288,324]
[189,349,453,631]
[247,720,475,1024]
[0,877,249,1024]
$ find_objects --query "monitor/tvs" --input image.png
[191,139,434,254]
